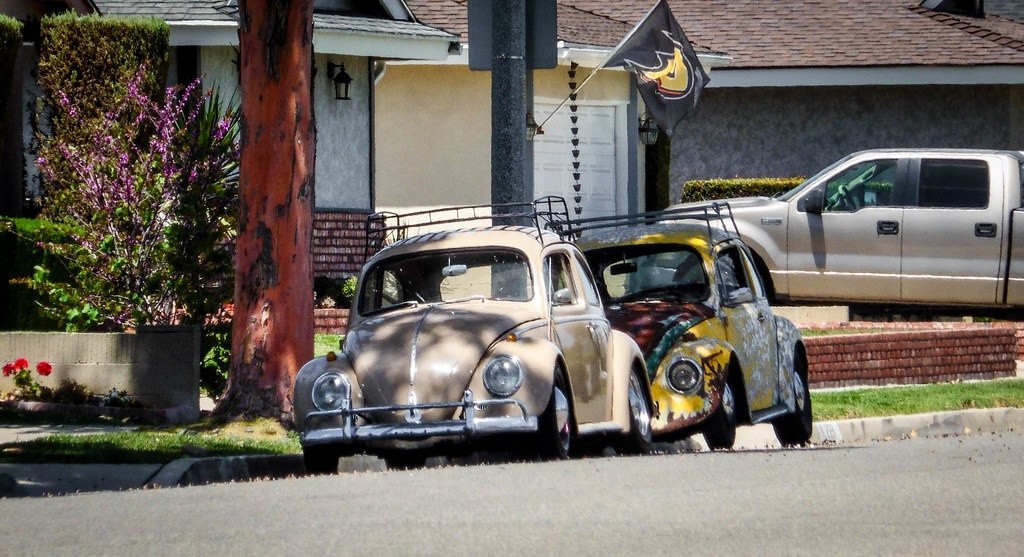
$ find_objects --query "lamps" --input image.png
[527,113,538,141]
[638,115,658,147]
[327,61,354,100]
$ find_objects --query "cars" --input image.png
[291,200,655,476]
[543,203,814,450]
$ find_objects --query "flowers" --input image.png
[2,359,52,401]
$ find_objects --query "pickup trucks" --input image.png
[654,148,1024,310]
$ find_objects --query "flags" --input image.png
[604,0,710,139]
